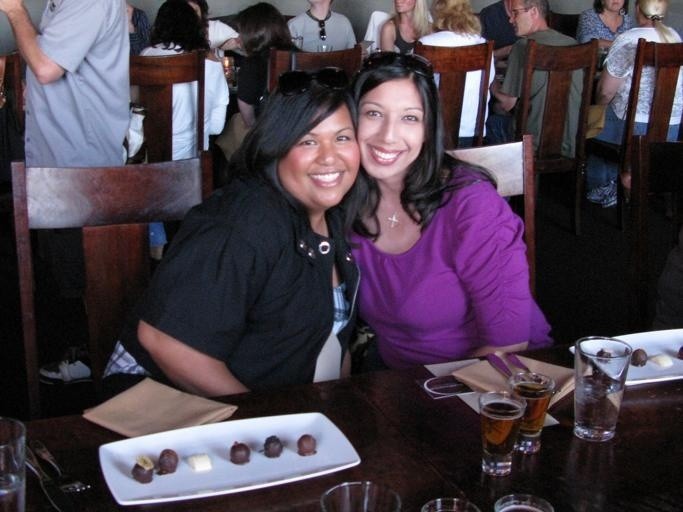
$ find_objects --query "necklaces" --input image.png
[388,206,403,227]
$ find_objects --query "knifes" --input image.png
[23,445,75,512]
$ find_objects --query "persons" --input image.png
[120,0,682,277]
[98,65,361,398]
[0,0,133,392]
[342,49,555,372]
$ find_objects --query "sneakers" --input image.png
[602,195,617,208]
[38,360,93,385]
[587,181,618,202]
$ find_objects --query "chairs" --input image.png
[0,54,25,128]
[268,44,361,94]
[631,134,681,328]
[442,133,537,299]
[483,39,598,235]
[121,49,205,163]
[9,152,213,420]
[587,38,682,230]
[413,39,494,152]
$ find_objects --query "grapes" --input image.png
[596,350,611,362]
[130,464,153,483]
[631,349,647,366]
[678,346,683,360]
[264,437,283,458]
[157,449,178,473]
[297,436,318,456]
[228,441,250,463]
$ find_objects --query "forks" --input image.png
[28,439,92,493]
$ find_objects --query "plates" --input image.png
[95,408,363,507]
[569,326,682,387]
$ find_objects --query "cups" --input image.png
[570,335,633,444]
[477,389,528,478]
[289,36,303,50]
[315,44,332,53]
[506,370,557,455]
[221,56,234,87]
[317,481,555,511]
[0,417,25,511]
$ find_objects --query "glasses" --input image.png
[276,67,350,97]
[511,8,528,19]
[363,51,434,78]
